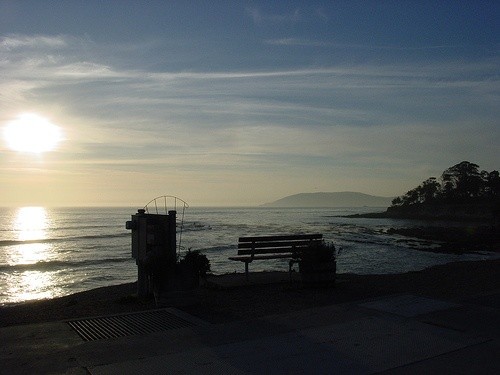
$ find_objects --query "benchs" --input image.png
[228,233,323,283]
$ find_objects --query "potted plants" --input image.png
[301,241,337,281]
[179,248,210,285]
